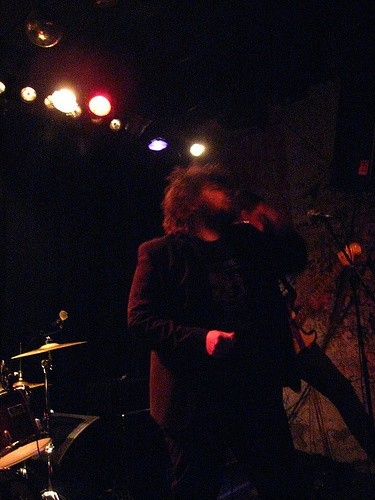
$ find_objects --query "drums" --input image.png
[0,437,66,500]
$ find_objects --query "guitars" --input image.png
[290,243,362,354]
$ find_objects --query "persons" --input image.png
[229,187,374,462]
[124,156,301,500]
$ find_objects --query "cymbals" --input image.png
[0,382,51,396]
[11,341,87,360]
